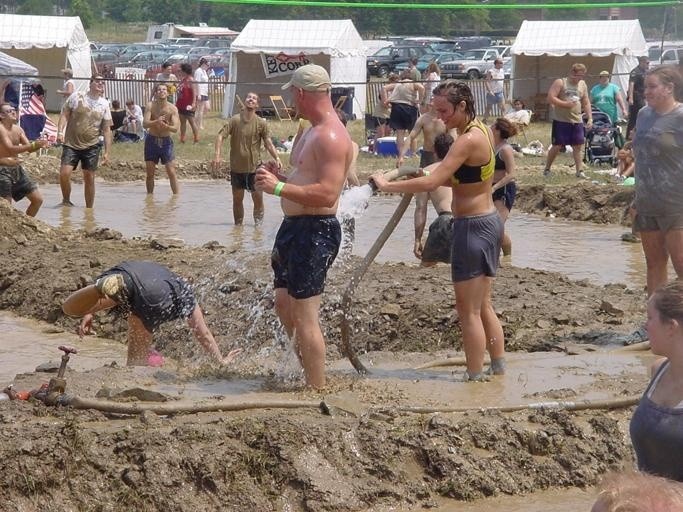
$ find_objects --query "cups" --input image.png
[3,109,17,114]
[95,80,105,85]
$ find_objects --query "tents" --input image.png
[0,14,93,112]
[508,20,647,122]
[221,19,367,120]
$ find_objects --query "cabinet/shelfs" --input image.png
[281,139,285,145]
[39,133,43,141]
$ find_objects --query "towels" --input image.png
[330,87,356,121]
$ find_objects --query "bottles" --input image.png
[281,64,331,92]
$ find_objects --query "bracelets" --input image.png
[58,132,63,136]
[274,182,286,196]
[32,142,35,149]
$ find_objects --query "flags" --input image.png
[20,81,58,137]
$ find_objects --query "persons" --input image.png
[369,78,507,381]
[589,57,651,180]
[1,104,48,218]
[58,68,77,98]
[623,65,683,346]
[142,84,181,193]
[334,108,369,251]
[372,58,442,153]
[56,75,114,208]
[629,280,683,484]
[214,92,282,225]
[590,467,683,512]
[62,261,242,367]
[543,64,592,181]
[109,100,144,135]
[396,94,458,168]
[253,64,353,394]
[481,59,530,128]
[150,57,211,145]
[490,117,517,257]
[413,132,455,267]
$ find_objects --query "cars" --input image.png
[186,105,193,111]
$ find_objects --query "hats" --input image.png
[88,37,231,78]
[363,35,513,81]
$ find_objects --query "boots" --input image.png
[645,42,683,68]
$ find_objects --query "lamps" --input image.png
[516,109,532,146]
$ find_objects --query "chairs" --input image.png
[94,274,125,297]
[575,169,591,180]
[543,169,553,177]
[623,322,658,346]
[614,174,627,184]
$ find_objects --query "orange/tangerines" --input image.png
[577,106,621,168]
